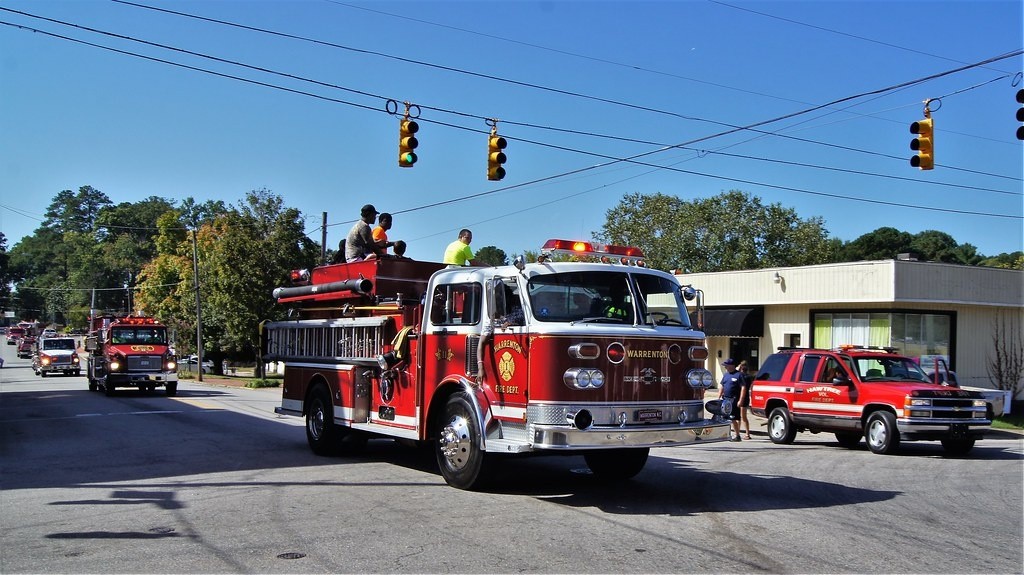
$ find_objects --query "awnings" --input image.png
[688,307,764,339]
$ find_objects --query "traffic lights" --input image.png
[910,118,933,170]
[1016,90,1024,141]
[399,120,418,167]
[488,136,506,181]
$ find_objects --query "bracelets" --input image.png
[477,361,484,364]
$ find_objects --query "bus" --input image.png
[0,316,22,334]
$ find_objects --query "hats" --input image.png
[721,360,736,367]
[362,205,380,214]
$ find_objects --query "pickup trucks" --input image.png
[41,328,58,338]
[750,342,994,457]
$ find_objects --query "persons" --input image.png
[717,358,746,442]
[834,366,850,383]
[444,228,492,267]
[394,239,414,261]
[371,212,393,254]
[734,360,754,440]
[476,293,526,385]
[344,205,387,263]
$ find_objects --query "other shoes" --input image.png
[743,435,751,440]
[731,436,741,442]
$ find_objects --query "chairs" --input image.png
[866,369,883,379]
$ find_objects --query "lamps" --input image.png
[773,272,783,283]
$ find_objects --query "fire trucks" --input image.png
[7,321,50,345]
[259,236,716,493]
[85,315,178,397]
[31,338,81,377]
[17,336,39,359]
